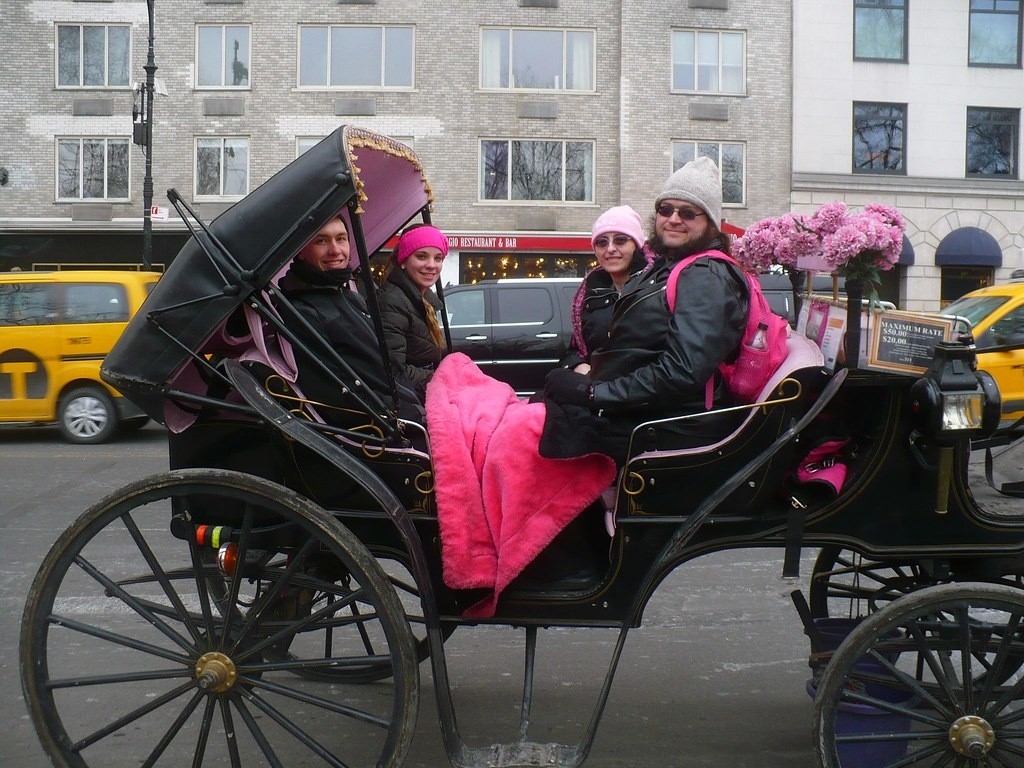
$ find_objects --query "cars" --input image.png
[936,284,1024,420]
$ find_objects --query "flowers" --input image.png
[730,201,906,316]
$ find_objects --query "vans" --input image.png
[428,271,881,402]
[0,271,165,445]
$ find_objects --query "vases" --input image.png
[788,273,806,324]
[843,281,862,369]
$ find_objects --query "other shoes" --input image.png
[514,560,598,591]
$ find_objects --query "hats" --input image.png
[655,156,722,233]
[591,205,645,249]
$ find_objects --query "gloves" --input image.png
[543,367,592,408]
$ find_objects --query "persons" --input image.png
[539,156,751,589]
[286,219,428,441]
[379,224,450,386]
[561,205,664,383]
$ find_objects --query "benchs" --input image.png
[238,336,436,497]
[601,325,825,538]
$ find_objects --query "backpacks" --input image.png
[666,251,788,411]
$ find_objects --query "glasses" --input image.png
[592,234,630,248]
[656,205,706,221]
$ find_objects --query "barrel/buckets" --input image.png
[805,662,917,768]
[807,616,910,686]
